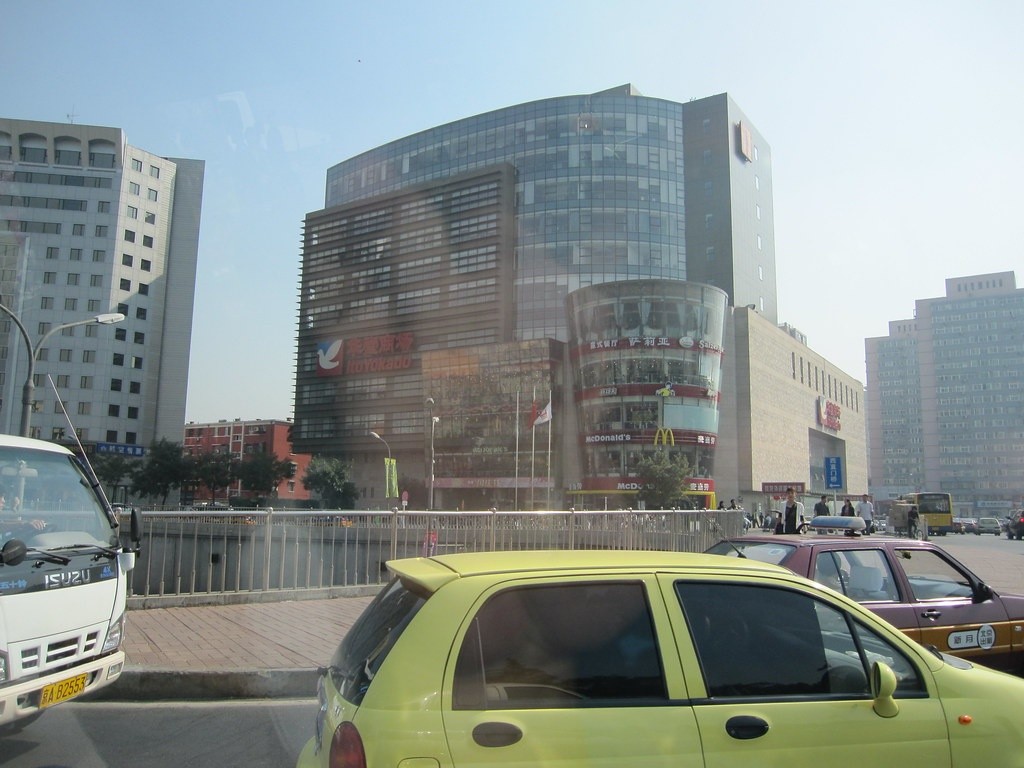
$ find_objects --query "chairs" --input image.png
[841,565,891,603]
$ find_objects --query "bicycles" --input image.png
[900,520,923,541]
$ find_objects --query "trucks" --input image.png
[0,434,142,735]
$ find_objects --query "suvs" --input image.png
[1005,509,1024,540]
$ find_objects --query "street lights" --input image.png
[423,398,436,511]
[368,432,390,511]
[0,302,126,509]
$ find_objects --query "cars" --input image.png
[870,519,886,531]
[295,548,1024,768]
[108,503,141,511]
[703,516,1024,678]
[952,518,1009,536]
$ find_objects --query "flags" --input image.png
[528,398,537,429]
[534,401,552,425]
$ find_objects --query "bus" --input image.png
[890,491,954,536]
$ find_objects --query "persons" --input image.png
[717,486,875,536]
[817,551,842,590]
[909,506,921,539]
